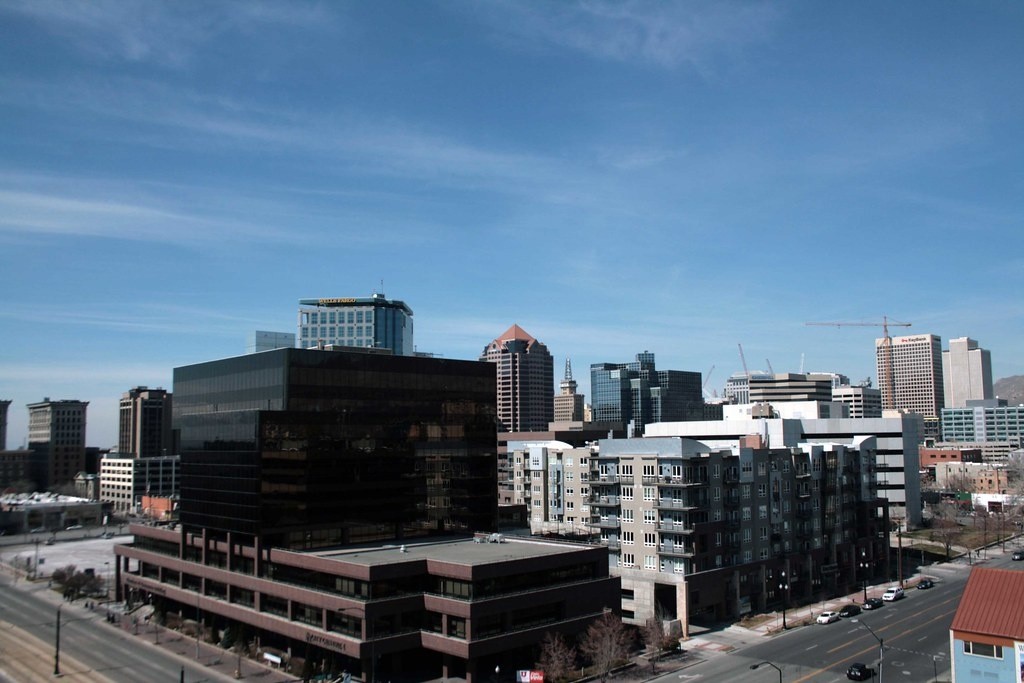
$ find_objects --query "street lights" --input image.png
[851,618,884,683]
[104,561,110,620]
[859,552,870,602]
[779,572,789,629]
[750,661,782,683]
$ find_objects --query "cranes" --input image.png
[766,358,776,374]
[804,314,912,410]
[738,343,749,376]
[703,365,715,391]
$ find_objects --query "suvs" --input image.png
[883,585,904,602]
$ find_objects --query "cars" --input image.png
[862,597,883,610]
[847,663,875,681]
[816,610,840,625]
[839,603,861,617]
[916,578,934,590]
[1012,548,1024,561]
